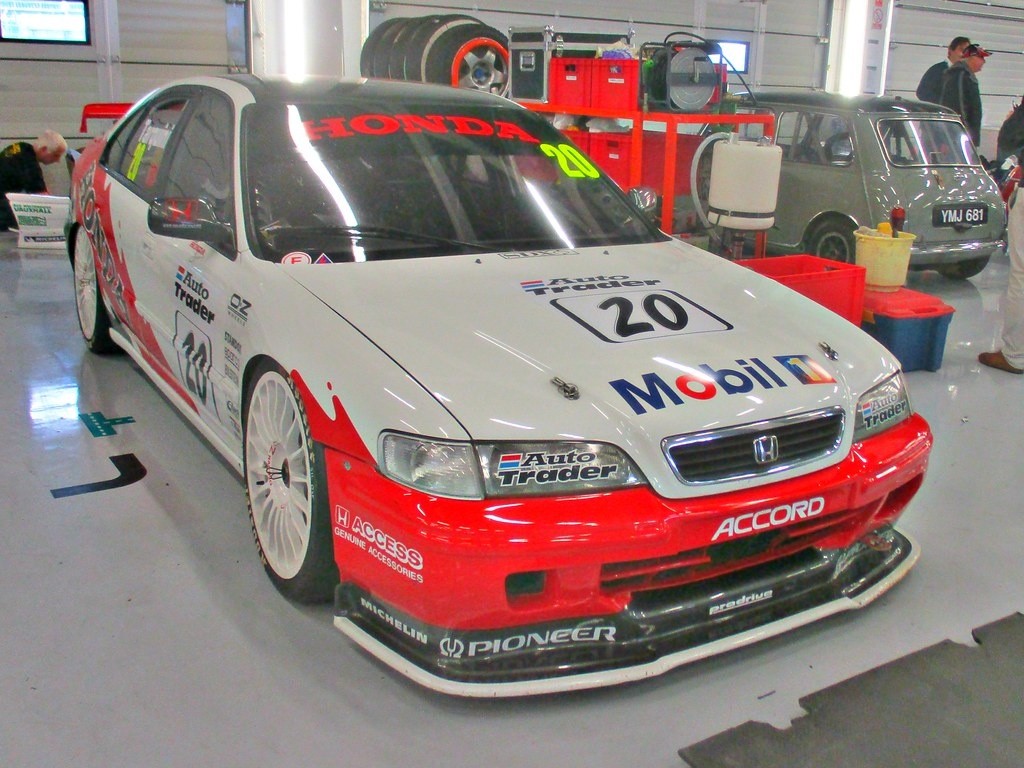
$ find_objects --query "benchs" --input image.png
[777,145,823,163]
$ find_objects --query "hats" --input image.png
[962,44,993,58]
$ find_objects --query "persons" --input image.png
[978,95,1024,374]
[0,130,67,233]
[916,37,993,157]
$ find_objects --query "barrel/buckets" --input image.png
[853,229,917,292]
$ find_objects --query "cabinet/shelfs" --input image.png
[522,101,775,261]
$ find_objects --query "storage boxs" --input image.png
[862,288,956,374]
[731,254,865,329]
[6,193,72,250]
[506,27,637,102]
[590,132,706,196]
[550,58,638,109]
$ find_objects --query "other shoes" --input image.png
[978,350,1023,374]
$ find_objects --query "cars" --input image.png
[64,77,935,699]
[699,86,1008,282]
